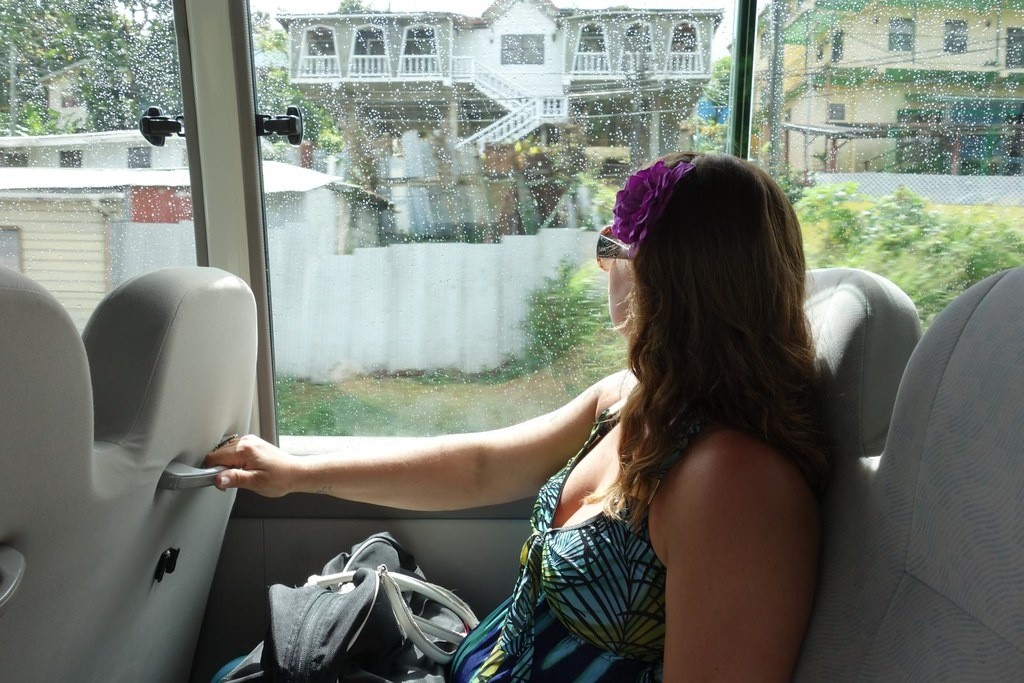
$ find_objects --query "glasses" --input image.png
[596,226,633,271]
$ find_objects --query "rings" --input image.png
[214,434,238,450]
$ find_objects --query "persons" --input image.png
[202,152,832,683]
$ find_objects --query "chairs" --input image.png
[765,260,1024,683]
[1,264,260,683]
